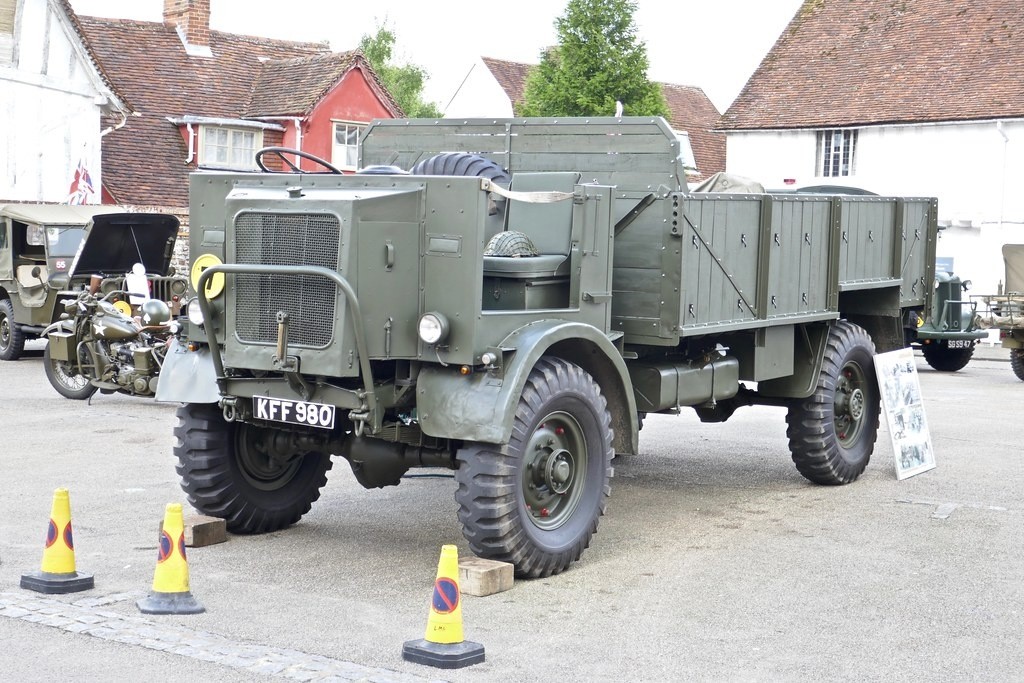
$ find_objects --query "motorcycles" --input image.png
[44,275,195,406]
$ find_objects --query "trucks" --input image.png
[903,257,987,373]
[152,116,947,580]
[970,244,1023,378]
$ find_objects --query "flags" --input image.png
[69,143,95,205]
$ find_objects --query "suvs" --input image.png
[1,204,190,361]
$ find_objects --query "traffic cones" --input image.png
[400,543,487,669]
[133,504,207,615]
[16,487,95,597]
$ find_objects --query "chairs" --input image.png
[408,152,512,252]
[482,173,583,281]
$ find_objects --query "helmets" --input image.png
[140,299,170,325]
[483,230,539,256]
